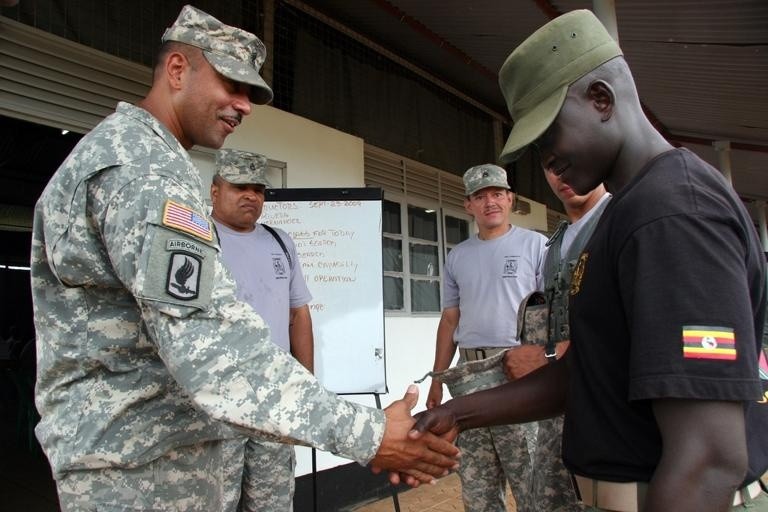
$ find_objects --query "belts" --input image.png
[569,472,762,512]
[459,348,500,361]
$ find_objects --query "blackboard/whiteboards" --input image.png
[255,187,387,394]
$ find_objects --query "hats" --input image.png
[462,163,511,196]
[498,9,624,164]
[160,4,275,105]
[215,148,273,189]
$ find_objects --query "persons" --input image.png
[425,163,581,511]
[370,6,768,511]
[30,6,460,512]
[542,166,614,509]
[209,146,313,511]
